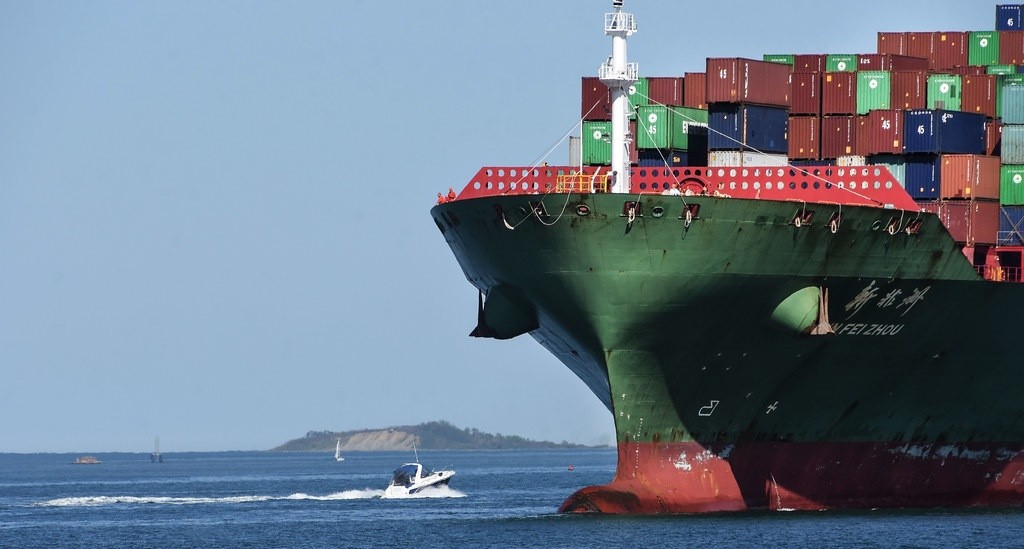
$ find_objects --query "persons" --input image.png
[435,187,457,205]
[662,184,720,197]
[984,264,1006,282]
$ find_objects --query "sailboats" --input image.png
[334,437,345,462]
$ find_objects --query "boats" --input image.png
[71,455,101,464]
[384,437,456,501]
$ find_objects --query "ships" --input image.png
[430,3,1024,518]
